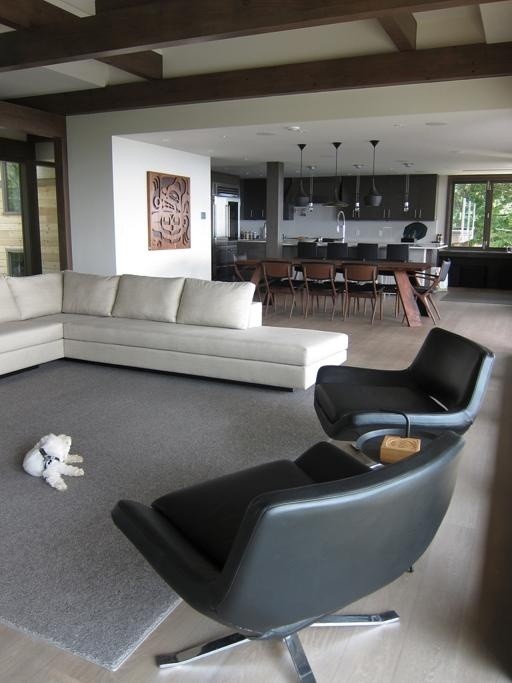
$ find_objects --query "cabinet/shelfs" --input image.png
[283,177,293,223]
[371,172,402,220]
[294,175,338,206]
[398,172,440,222]
[239,176,268,222]
[337,176,372,220]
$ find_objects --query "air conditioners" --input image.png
[212,179,242,199]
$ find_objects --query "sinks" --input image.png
[315,237,340,243]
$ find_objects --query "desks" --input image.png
[356,426,446,573]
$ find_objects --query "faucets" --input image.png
[336,210,346,239]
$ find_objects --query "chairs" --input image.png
[222,232,451,328]
[307,321,497,468]
[110,423,468,682]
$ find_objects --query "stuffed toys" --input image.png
[22,430,85,491]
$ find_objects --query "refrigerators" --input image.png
[210,194,241,241]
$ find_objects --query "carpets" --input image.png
[0,342,332,676]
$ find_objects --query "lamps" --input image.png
[365,136,385,210]
[294,138,311,209]
[322,138,347,210]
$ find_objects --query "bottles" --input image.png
[318,235,322,244]
[241,230,257,239]
[436,232,442,242]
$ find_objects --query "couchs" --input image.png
[0,267,351,396]
